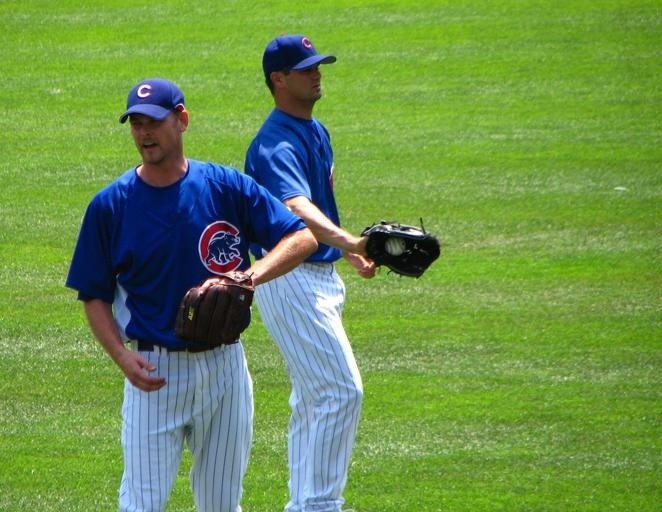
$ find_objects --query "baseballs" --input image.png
[386,236,406,255]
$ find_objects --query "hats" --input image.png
[118,78,188,126]
[261,31,340,80]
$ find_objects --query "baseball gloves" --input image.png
[362,220,440,279]
[177,271,254,346]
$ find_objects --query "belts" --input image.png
[128,333,230,353]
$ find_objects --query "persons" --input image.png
[65,80,319,511]
[244,32,443,512]
